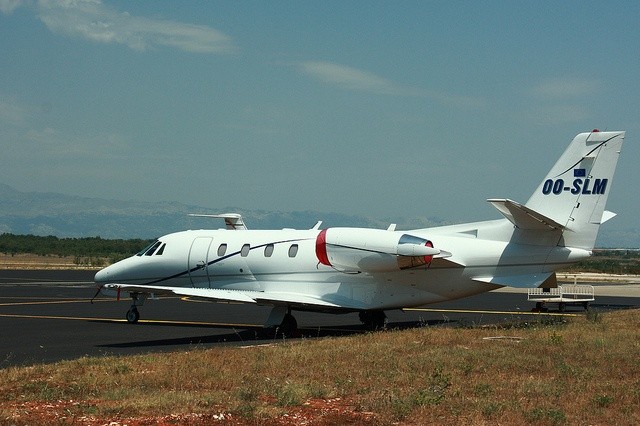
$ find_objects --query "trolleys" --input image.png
[528,284,594,312]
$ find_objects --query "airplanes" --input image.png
[94,129,625,338]
